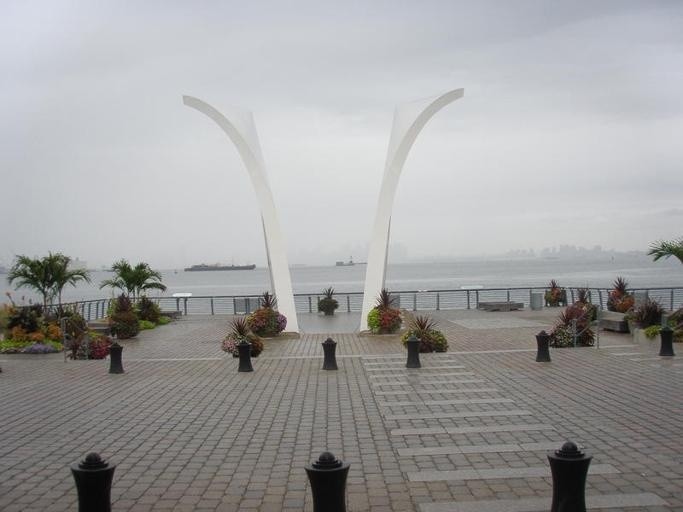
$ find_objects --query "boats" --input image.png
[335,255,367,266]
[183,262,257,271]
